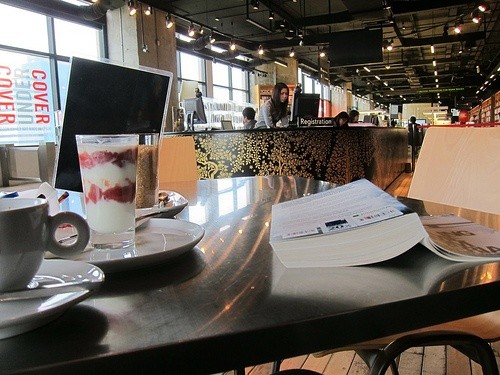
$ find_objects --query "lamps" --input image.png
[128,0,500,109]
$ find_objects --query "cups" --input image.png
[75,135,139,249]
[135,133,160,212]
[0,196,91,293]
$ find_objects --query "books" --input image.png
[269,178,500,269]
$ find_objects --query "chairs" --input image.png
[157,136,198,183]
[312,126,500,375]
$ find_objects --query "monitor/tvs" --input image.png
[330,29,383,68]
[290,93,320,122]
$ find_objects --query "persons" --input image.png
[349,110,360,123]
[242,107,257,129]
[408,116,423,133]
[254,82,290,128]
[334,111,349,126]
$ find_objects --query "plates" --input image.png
[135,190,189,216]
[42,218,204,273]
[0,259,105,341]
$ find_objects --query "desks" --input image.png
[0,174,500,375]
